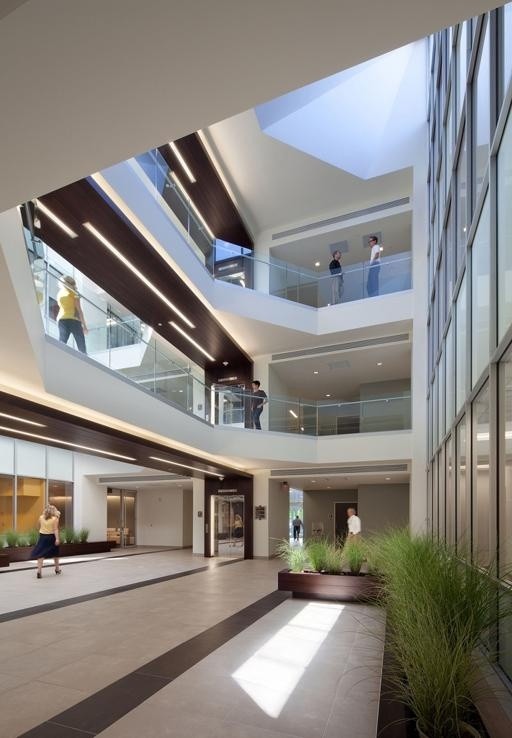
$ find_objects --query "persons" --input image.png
[292,515,304,542]
[56,275,90,357]
[29,504,62,578]
[328,250,345,305]
[340,507,365,560]
[247,380,269,431]
[366,235,381,298]
[230,514,244,547]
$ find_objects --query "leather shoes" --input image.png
[36,569,62,579]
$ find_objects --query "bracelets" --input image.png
[261,403,262,406]
